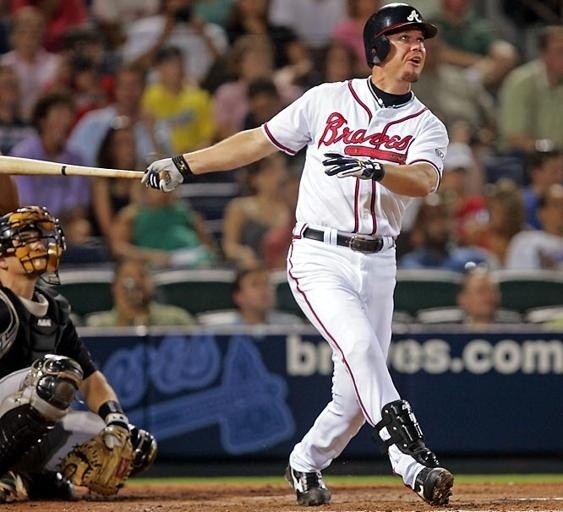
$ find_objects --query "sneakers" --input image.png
[0,470,31,503]
[414,467,454,506]
[285,463,331,507]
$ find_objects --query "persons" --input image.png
[1,0,561,327]
[1,204,160,506]
[140,3,456,507]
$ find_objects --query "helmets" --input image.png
[0,207,68,286]
[363,2,438,69]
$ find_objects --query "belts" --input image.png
[303,227,394,251]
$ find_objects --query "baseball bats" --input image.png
[0,157,170,186]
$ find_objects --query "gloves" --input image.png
[140,154,195,193]
[322,153,384,181]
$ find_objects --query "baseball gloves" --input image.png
[56,423,134,501]
